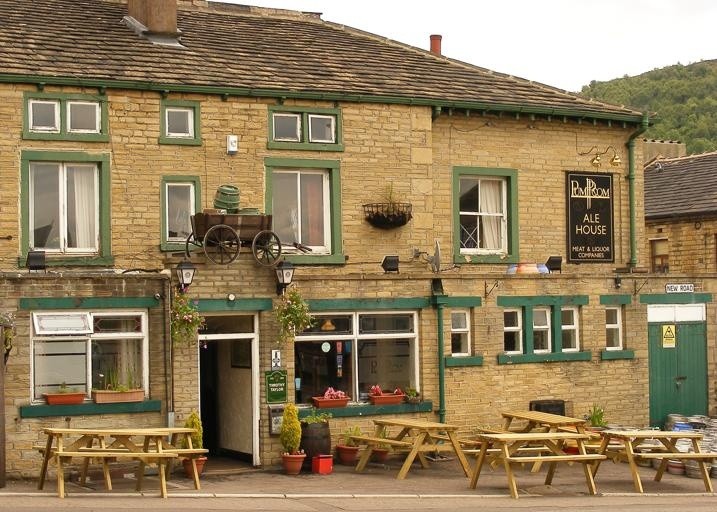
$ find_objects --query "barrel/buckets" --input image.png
[299,421,330,473]
[529,400,566,417]
[236,206,262,215]
[672,422,693,454]
[214,184,241,214]
[204,208,227,215]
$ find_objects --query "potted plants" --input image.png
[177,409,208,480]
[42,381,88,405]
[584,402,610,444]
[91,367,145,404]
[405,387,421,403]
[279,403,364,475]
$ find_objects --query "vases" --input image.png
[367,393,405,405]
[312,396,351,409]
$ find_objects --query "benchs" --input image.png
[541,423,603,465]
[458,447,552,454]
[477,425,526,467]
[78,448,209,490]
[580,443,667,453]
[476,426,515,433]
[504,453,607,464]
[637,452,716,461]
[31,442,171,490]
[351,435,412,453]
[429,432,482,446]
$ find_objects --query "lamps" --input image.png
[381,256,400,274]
[545,256,562,274]
[581,146,621,168]
[26,251,47,274]
[176,252,197,289]
[274,256,297,295]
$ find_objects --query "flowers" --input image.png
[323,387,345,400]
[169,286,208,350]
[370,384,402,396]
[274,283,318,344]
[0,312,16,353]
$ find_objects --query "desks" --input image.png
[38,426,199,499]
[502,411,587,474]
[356,418,472,480]
[469,433,597,498]
[601,429,714,493]
